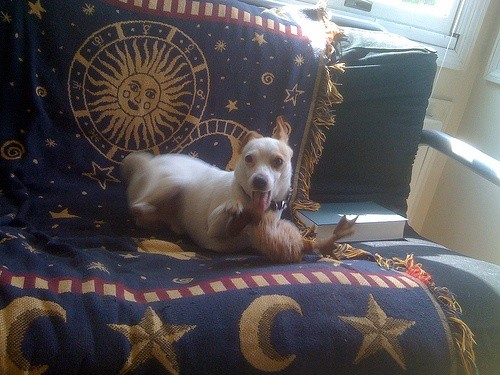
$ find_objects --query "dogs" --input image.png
[117,114,295,262]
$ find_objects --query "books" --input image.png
[295,201,410,243]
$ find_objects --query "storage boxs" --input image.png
[296,200,409,243]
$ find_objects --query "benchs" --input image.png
[0,0,500,375]
